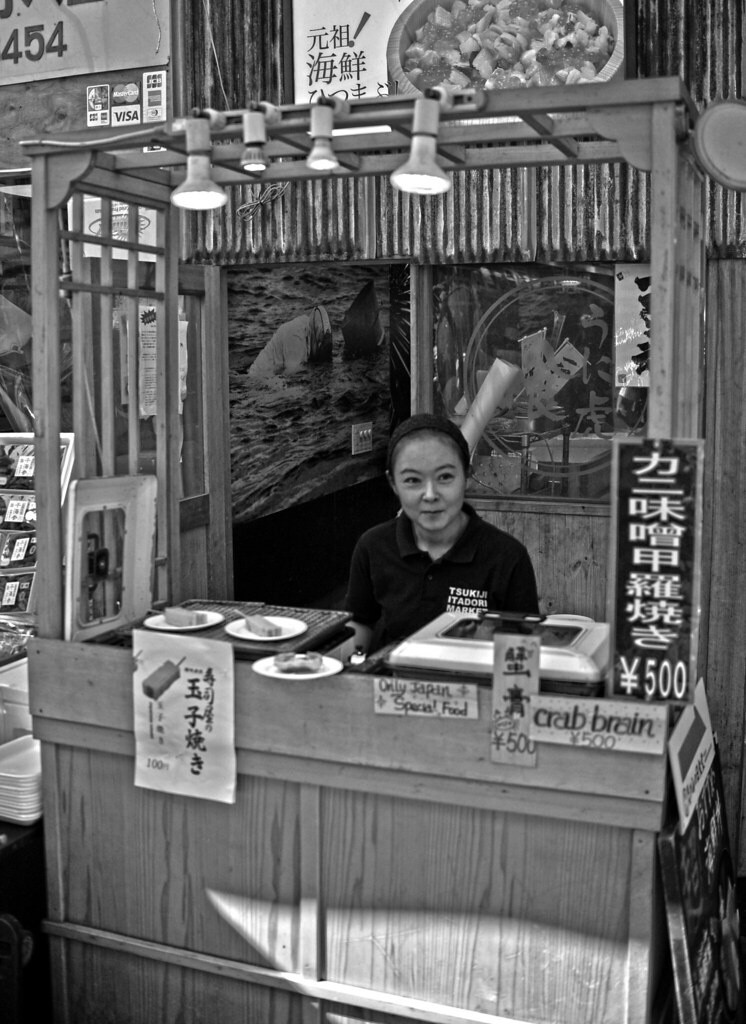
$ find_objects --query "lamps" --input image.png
[167,83,455,216]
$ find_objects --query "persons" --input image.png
[248,306,333,378]
[344,414,540,642]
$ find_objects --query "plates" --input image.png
[0,735,42,825]
[251,653,344,680]
[224,616,308,640]
[145,610,223,631]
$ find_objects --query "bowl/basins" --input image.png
[385,0,625,95]
